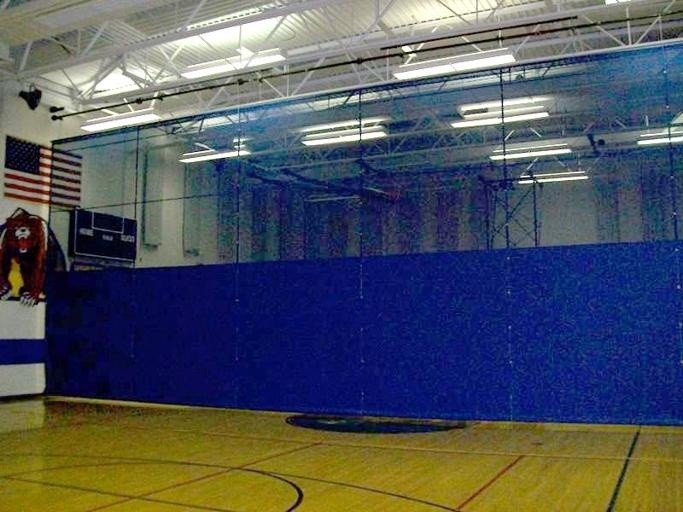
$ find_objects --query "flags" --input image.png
[3,134,84,212]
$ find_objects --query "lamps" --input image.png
[392,48,514,80]
[177,147,251,163]
[179,48,287,79]
[301,125,386,146]
[636,130,682,146]
[450,96,589,185]
[79,108,162,134]
[3,79,43,111]
